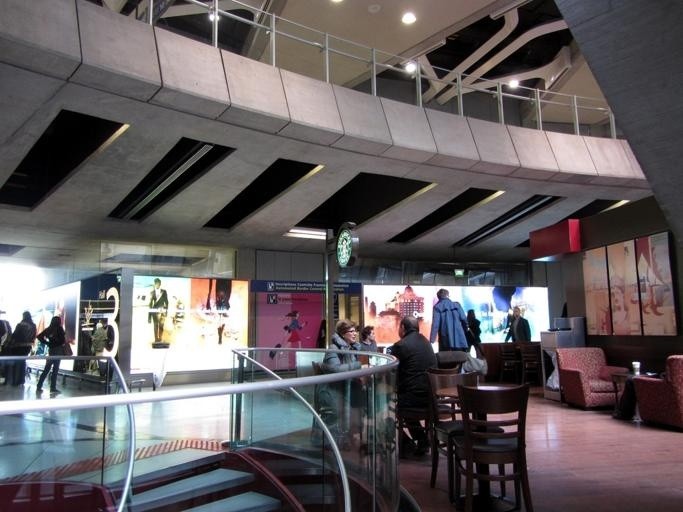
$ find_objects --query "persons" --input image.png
[35,315,66,393]
[429,289,474,369]
[311,321,362,433]
[388,316,438,461]
[360,326,378,353]
[505,307,532,342]
[11,311,36,385]
[317,320,326,348]
[206,279,232,344]
[93,323,111,377]
[464,310,482,352]
[148,278,169,342]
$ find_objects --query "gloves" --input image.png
[466,329,475,344]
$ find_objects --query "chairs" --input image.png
[426,364,534,512]
[312,361,321,427]
[632,355,683,428]
[555,347,629,411]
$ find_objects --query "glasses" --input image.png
[347,329,359,333]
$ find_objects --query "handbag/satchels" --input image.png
[63,342,73,356]
[1,332,10,347]
[463,350,488,375]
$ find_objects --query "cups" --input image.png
[632,362,640,376]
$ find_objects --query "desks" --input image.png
[611,374,660,420]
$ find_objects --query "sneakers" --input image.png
[399,442,429,460]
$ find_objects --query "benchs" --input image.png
[27,364,147,394]
